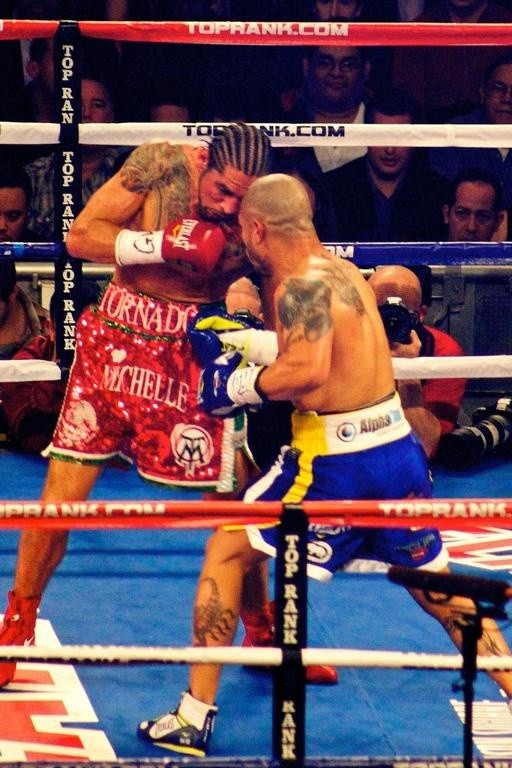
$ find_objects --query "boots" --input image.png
[239,600,338,684]
[0,589,41,687]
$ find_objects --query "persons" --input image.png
[137,167,512,758]
[0,123,339,686]
[0,1,511,474]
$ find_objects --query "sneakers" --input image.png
[136,689,221,759]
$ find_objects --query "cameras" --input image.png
[379,296,421,345]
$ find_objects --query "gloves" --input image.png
[187,308,279,368]
[115,217,227,280]
[196,366,268,418]
